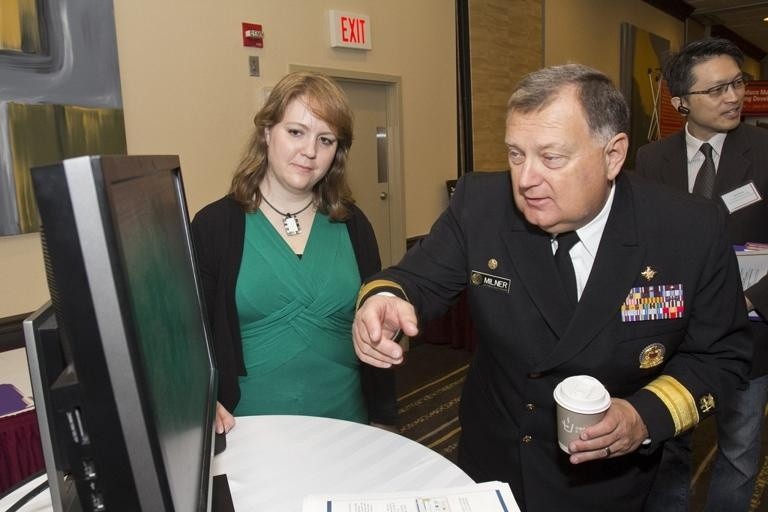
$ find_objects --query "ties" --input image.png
[553,230,578,313]
[693,143,717,201]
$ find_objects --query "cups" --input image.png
[552,374,612,456]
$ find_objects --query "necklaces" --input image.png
[254,184,316,235]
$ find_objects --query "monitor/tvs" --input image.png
[23,152,237,512]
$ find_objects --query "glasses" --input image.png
[680,73,750,98]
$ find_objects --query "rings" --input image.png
[603,445,614,457]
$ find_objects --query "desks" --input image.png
[0,413,505,512]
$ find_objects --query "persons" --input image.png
[189,70,404,436]
[632,36,767,512]
[350,60,754,512]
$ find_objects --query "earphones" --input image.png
[677,105,690,116]
